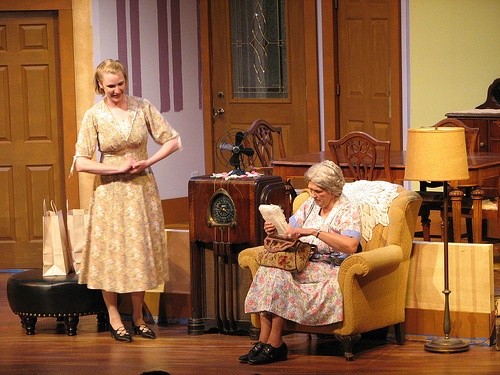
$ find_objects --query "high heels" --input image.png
[109,323,132,343]
[131,319,156,339]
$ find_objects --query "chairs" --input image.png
[328,131,391,183]
[249,119,287,177]
[414,118,484,244]
[238,179,422,363]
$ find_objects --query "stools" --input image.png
[7,269,122,336]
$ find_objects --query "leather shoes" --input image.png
[238,341,288,365]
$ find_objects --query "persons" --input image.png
[68,58,180,341]
[238,160,362,365]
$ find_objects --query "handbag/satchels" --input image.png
[42,198,71,276]
[66,198,89,275]
[256,234,318,273]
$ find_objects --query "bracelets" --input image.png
[315,229,322,238]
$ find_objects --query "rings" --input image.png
[288,235,291,236]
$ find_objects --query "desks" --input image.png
[188,172,297,334]
[269,150,500,244]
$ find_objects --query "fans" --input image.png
[216,128,257,178]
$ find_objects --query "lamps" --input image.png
[404,127,470,353]
